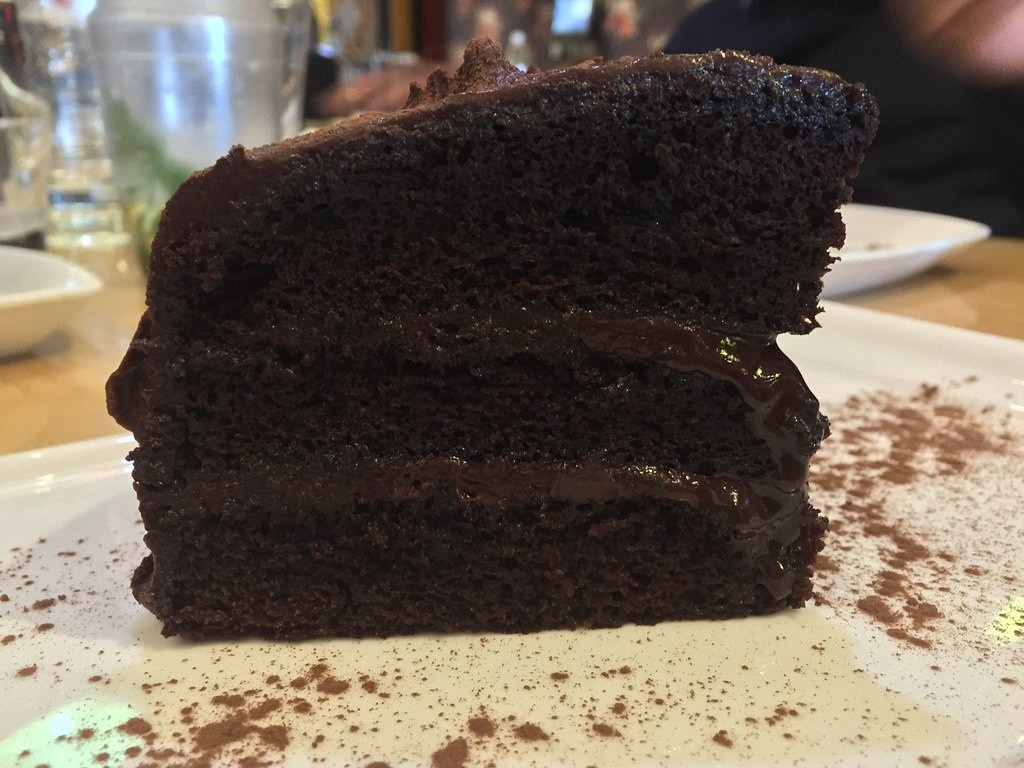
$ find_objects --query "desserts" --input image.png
[98,37,878,642]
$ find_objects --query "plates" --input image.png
[0,299,1024,765]
[817,202,993,299]
[1,244,102,359]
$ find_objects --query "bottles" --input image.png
[0,3,308,289]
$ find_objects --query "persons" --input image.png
[302,0,648,121]
[650,0,1024,242]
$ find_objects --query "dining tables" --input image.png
[0,238,1023,455]
